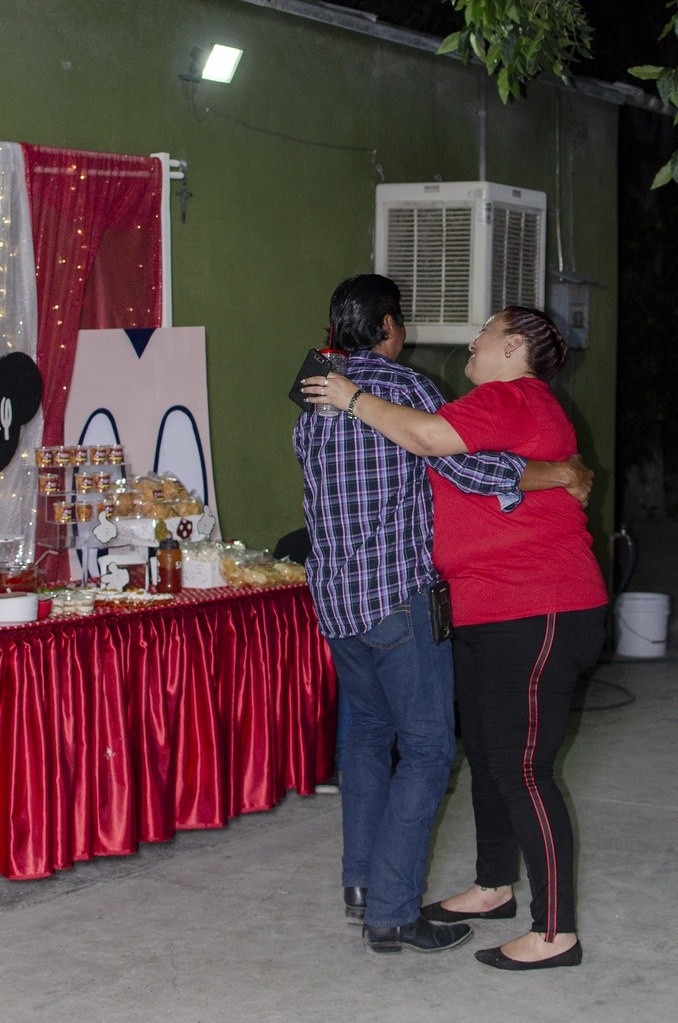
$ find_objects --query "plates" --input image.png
[1,592,38,626]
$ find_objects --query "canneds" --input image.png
[314,349,348,418]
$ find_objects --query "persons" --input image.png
[300,306,609,971]
[291,274,594,955]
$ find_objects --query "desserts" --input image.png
[40,479,305,613]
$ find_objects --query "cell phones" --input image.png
[289,348,331,411]
[429,580,454,643]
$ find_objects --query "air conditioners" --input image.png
[378,180,555,348]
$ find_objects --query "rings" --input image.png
[324,378,327,387]
[321,387,324,396]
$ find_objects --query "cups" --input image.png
[35,443,126,524]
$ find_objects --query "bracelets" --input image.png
[347,389,367,420]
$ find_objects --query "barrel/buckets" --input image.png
[613,592,670,656]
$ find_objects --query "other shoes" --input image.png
[420,894,518,923]
[473,939,582,971]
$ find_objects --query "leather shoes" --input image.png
[343,886,372,925]
[362,908,472,957]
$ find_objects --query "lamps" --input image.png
[175,38,245,86]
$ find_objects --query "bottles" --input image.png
[156,538,182,593]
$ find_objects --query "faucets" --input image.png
[615,523,632,546]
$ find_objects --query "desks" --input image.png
[0,552,342,885]
